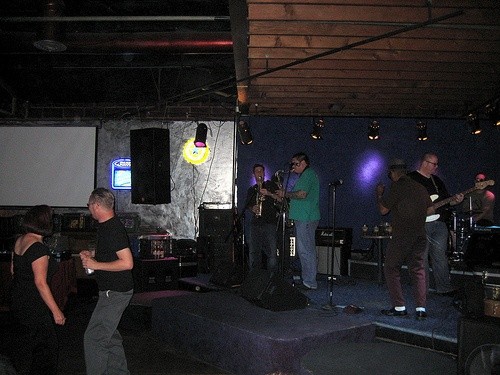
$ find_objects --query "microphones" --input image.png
[328,179,344,187]
[288,162,300,166]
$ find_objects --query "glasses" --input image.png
[425,160,440,167]
[87,203,95,207]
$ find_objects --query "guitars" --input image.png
[426,180,495,215]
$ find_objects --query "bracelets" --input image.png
[271,193,274,197]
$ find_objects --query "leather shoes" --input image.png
[416,311,427,321]
[381,306,409,316]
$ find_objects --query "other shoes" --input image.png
[291,280,310,290]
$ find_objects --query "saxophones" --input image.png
[255,176,265,217]
[275,170,294,227]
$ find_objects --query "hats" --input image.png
[387,158,408,172]
[476,174,486,181]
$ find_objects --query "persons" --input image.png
[377,159,429,321]
[10,205,65,375]
[407,153,465,296]
[246,164,281,278]
[79,188,134,375]
[461,174,496,272]
[275,153,321,290]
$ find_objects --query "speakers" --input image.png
[314,226,352,277]
[130,128,171,206]
[197,209,240,282]
